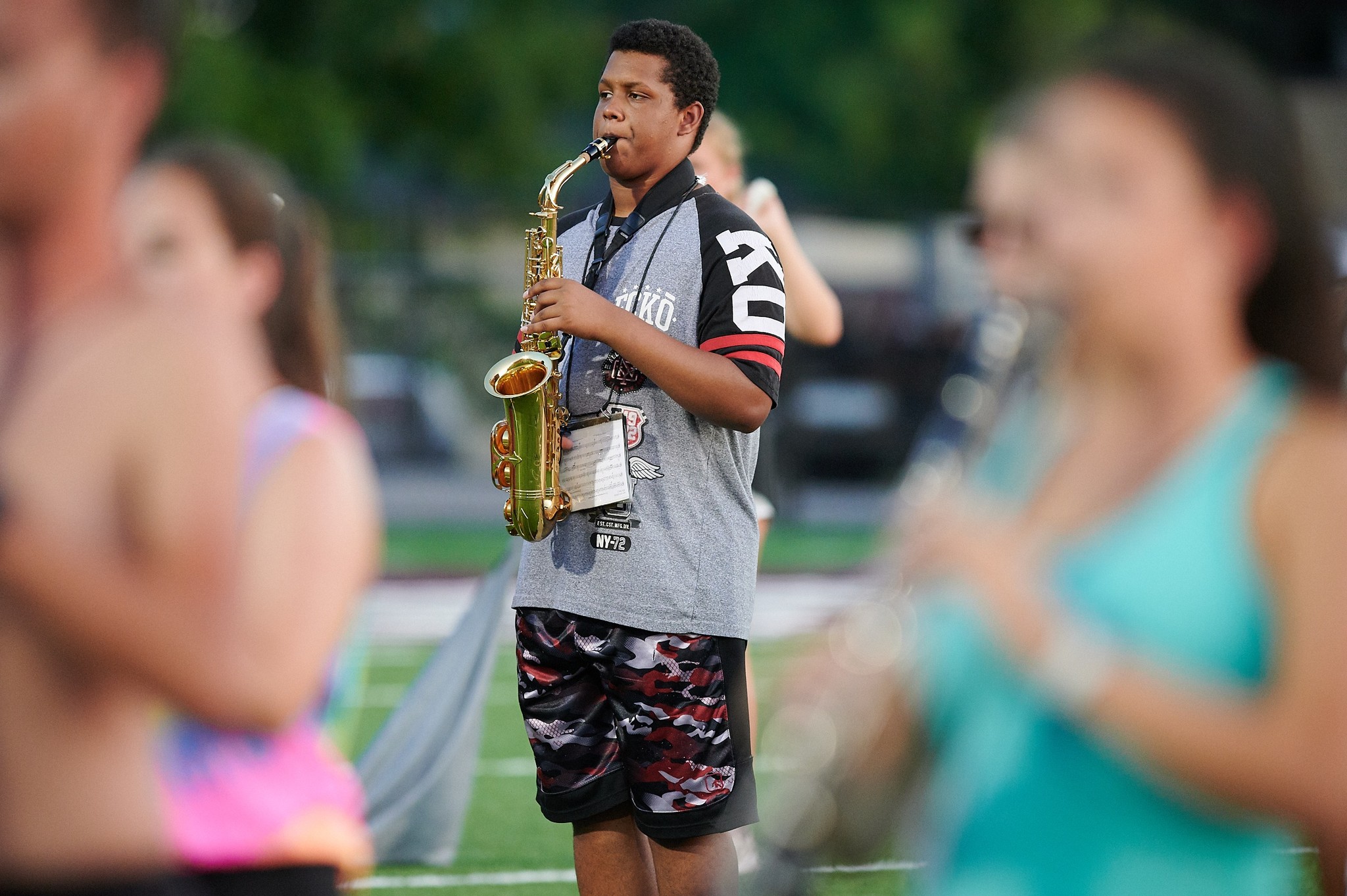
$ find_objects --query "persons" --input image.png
[112,132,379,896]
[0,0,244,896]
[510,19,787,896]
[687,110,841,874]
[777,34,1347,896]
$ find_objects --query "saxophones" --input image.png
[483,131,618,542]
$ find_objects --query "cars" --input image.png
[753,285,972,512]
